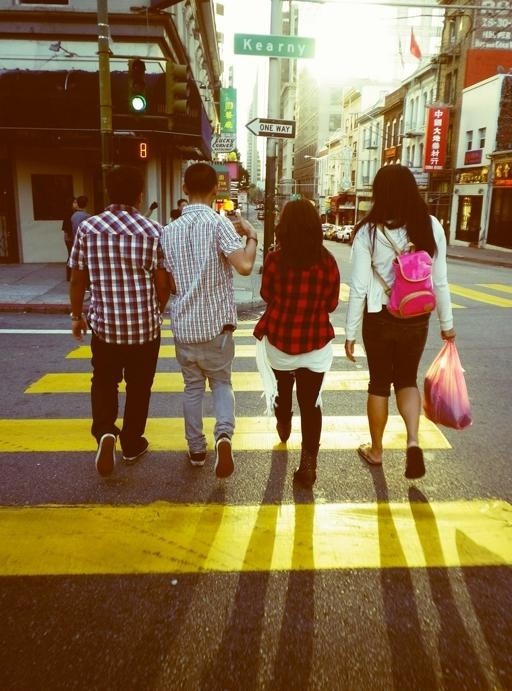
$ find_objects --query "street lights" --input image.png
[282,176,298,196]
[304,153,329,223]
[333,124,362,224]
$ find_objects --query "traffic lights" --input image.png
[163,56,196,122]
[126,57,147,115]
[108,133,151,165]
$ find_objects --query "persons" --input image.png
[160,163,257,478]
[253,199,340,491]
[345,164,456,479]
[61,195,189,293]
[69,166,171,478]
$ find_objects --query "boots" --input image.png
[273,407,294,445]
[291,444,321,485]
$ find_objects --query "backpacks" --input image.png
[368,220,435,319]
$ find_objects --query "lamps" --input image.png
[200,95,211,101]
[195,80,206,89]
[49,41,72,57]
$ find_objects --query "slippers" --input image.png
[404,446,426,481]
[356,440,384,469]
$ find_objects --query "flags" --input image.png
[410,29,422,60]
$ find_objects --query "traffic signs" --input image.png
[234,32,315,58]
[246,117,296,139]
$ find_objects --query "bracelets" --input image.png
[70,311,85,321]
[246,237,258,246]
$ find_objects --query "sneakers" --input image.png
[121,436,149,463]
[93,432,117,477]
[185,447,208,466]
[215,434,236,482]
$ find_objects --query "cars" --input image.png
[256,211,265,221]
[321,222,357,244]
